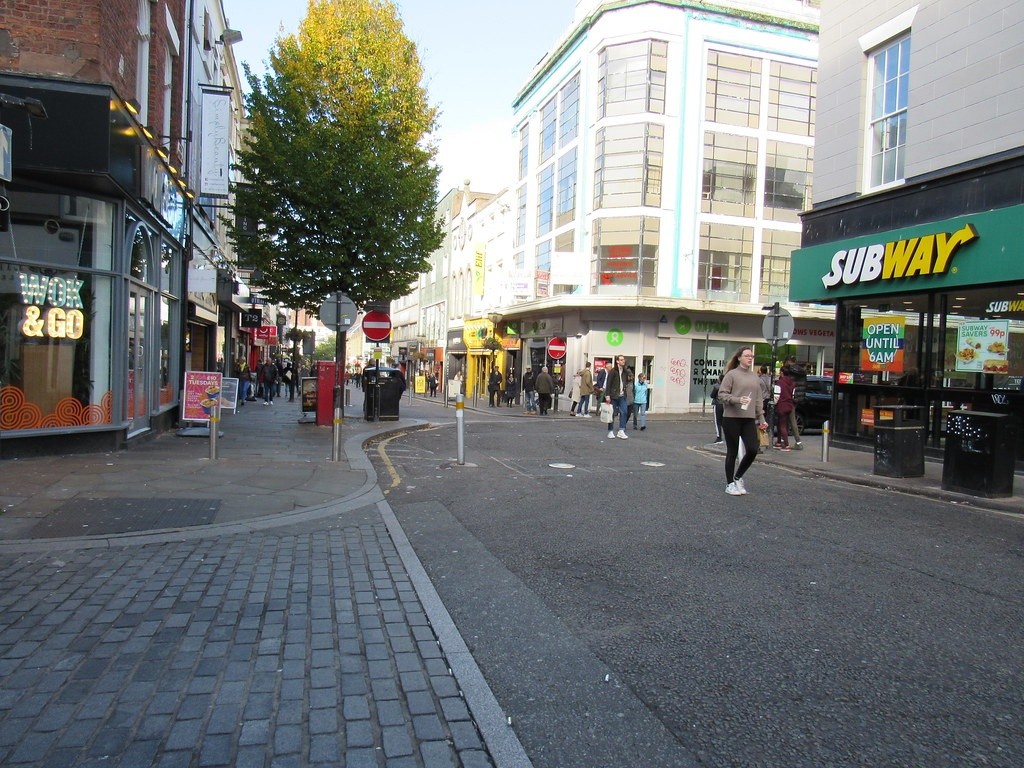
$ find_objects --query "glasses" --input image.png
[743,354,754,359]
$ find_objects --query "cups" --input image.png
[740,396,752,411]
[872,375,878,384]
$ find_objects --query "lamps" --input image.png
[186,188,196,200]
[195,244,237,284]
[168,164,181,172]
[122,98,142,114]
[139,125,157,138]
[179,177,190,187]
[158,146,170,159]
[215,29,243,49]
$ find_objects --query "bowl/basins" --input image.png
[200,398,218,414]
[205,386,220,399]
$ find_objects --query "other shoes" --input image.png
[607,431,615,439]
[781,442,784,448]
[531,410,537,415]
[713,438,723,444]
[570,414,575,416]
[576,413,584,417]
[781,446,791,451]
[641,426,646,430]
[523,410,531,414]
[791,442,803,450]
[584,414,592,418]
[617,430,628,439]
[773,444,782,448]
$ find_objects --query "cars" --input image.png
[766,375,836,435]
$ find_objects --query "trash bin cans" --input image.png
[874,405,927,480]
[363,366,399,421]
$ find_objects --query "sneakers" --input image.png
[734,477,746,495]
[725,482,741,496]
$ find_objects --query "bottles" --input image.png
[810,364,814,376]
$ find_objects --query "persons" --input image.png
[258,357,278,406]
[522,365,537,414]
[569,369,581,416]
[345,369,361,388]
[489,366,503,407]
[454,371,464,381]
[595,361,612,416]
[255,359,309,396]
[234,356,252,406]
[626,369,634,423]
[633,373,648,431]
[718,346,766,495]
[505,374,516,407]
[575,362,594,418]
[774,367,793,452]
[710,375,725,445]
[757,364,771,416]
[429,373,437,397]
[535,367,554,415]
[785,354,806,450]
[362,358,376,411]
[606,355,629,439]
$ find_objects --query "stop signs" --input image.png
[362,310,392,342]
[548,337,567,359]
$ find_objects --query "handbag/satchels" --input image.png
[592,384,602,397]
[600,401,613,423]
